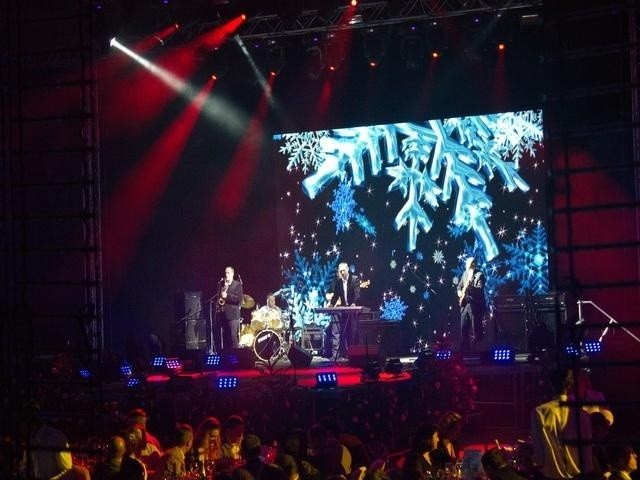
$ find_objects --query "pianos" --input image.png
[314,307,362,314]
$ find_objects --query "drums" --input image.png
[254,330,280,361]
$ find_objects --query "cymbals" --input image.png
[240,294,256,309]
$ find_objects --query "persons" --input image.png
[455,256,487,343]
[211,264,244,354]
[0,361,639,478]
[255,291,283,332]
[325,261,362,361]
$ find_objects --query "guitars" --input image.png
[459,267,478,306]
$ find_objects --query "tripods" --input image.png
[520,301,540,353]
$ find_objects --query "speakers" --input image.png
[168,290,206,350]
[287,346,313,367]
[348,344,386,368]
[356,319,402,356]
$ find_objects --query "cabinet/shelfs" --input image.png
[325,310,363,364]
[355,322,399,348]
[494,308,567,353]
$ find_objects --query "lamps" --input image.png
[437,350,455,360]
[494,347,510,362]
[153,357,187,370]
[120,361,133,376]
[207,354,221,368]
[568,337,603,359]
[127,376,140,392]
[80,368,89,377]
[315,371,338,389]
[219,376,240,390]
